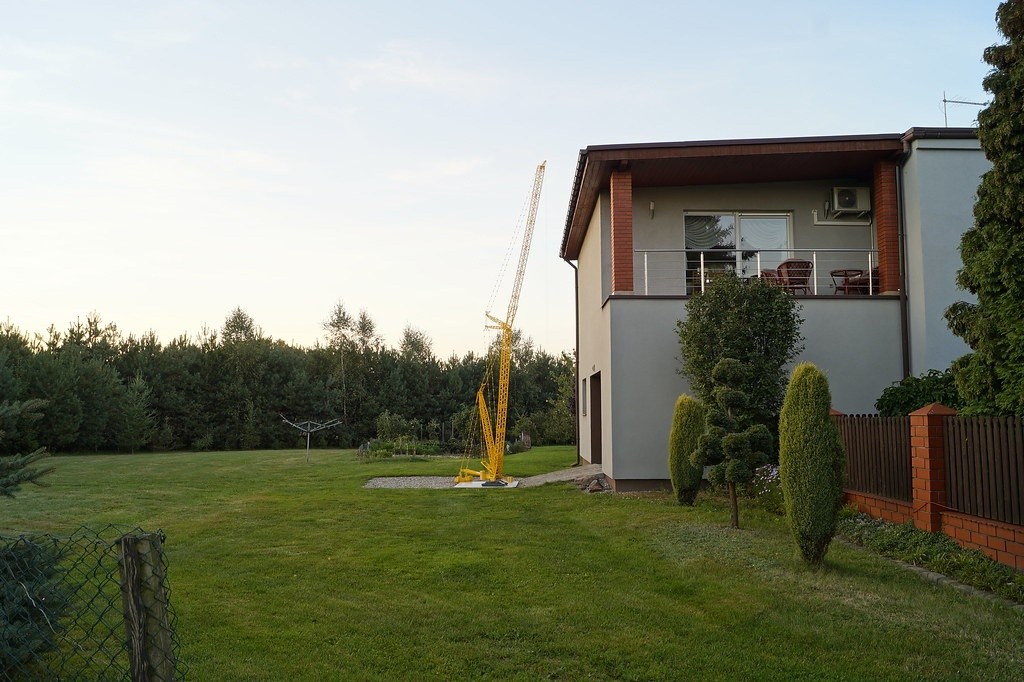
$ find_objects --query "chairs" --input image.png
[775,259,814,295]
[761,271,778,290]
[843,267,881,295]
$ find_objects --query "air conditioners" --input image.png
[830,186,871,214]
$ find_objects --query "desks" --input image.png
[830,268,862,295]
